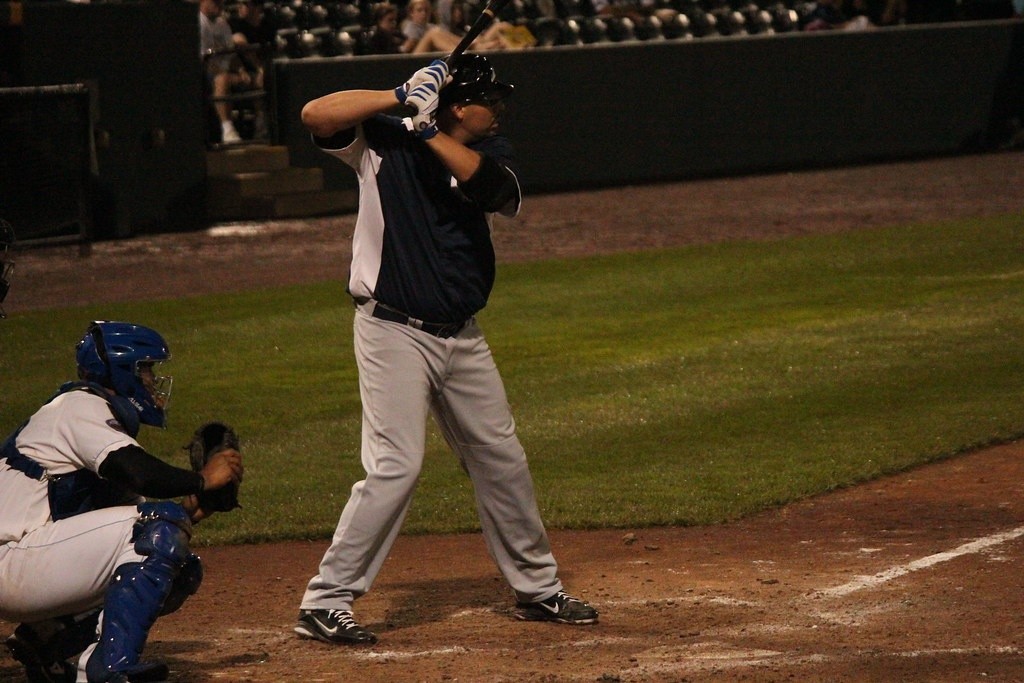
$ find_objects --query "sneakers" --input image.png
[6,627,77,683]
[88,658,169,683]
[514,588,599,624]
[295,605,377,644]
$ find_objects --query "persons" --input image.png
[1,320,244,683]
[198,0,874,143]
[292,52,598,652]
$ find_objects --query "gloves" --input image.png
[402,81,440,139]
[394,60,449,104]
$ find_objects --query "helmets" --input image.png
[76,319,173,429]
[440,52,515,102]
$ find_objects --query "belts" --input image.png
[357,294,478,339]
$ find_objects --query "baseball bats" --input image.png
[404,0,515,116]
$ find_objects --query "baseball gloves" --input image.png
[187,421,243,511]
